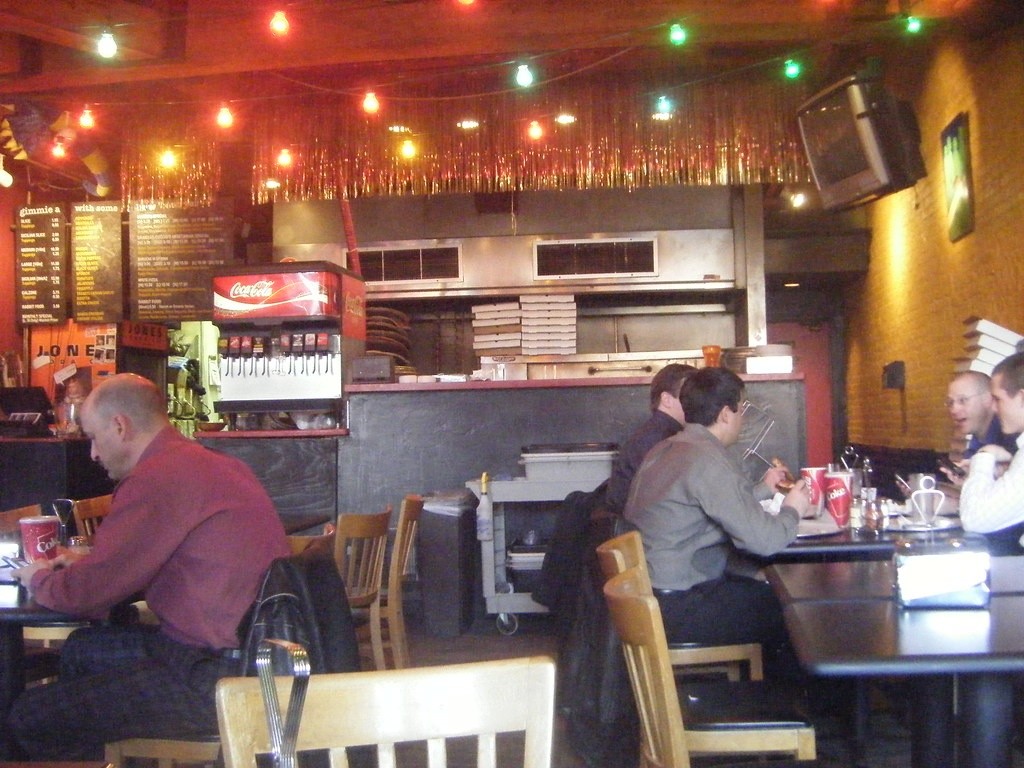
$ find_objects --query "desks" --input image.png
[0,513,331,764]
[782,594,1024,768]
[765,555,1024,768]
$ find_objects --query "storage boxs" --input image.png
[522,340,577,349]
[476,347,522,357]
[522,310,576,317]
[519,295,575,303]
[474,324,522,335]
[474,333,521,342]
[471,302,520,313]
[471,317,520,327]
[522,333,576,340]
[472,340,521,350]
[522,347,577,355]
[518,442,621,479]
[522,317,576,326]
[521,302,577,311]
[475,310,522,319]
[522,325,576,333]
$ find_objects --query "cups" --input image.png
[825,463,841,472]
[799,467,826,517]
[909,473,937,527]
[823,472,854,528]
[15,515,60,564]
[702,345,721,367]
[842,468,863,496]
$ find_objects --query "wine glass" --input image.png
[51,499,76,545]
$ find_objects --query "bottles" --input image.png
[66,535,90,555]
[476,471,494,541]
[850,497,889,534]
[892,540,919,565]
[940,538,971,553]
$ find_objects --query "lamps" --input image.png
[554,99,577,124]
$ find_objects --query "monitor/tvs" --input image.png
[795,70,918,211]
[0,386,60,424]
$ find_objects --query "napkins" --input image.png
[761,491,786,515]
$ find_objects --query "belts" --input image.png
[218,648,243,660]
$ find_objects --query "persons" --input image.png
[938,352,1024,547]
[606,363,698,511]
[11,373,293,760]
[623,367,812,669]
[895,370,1020,514]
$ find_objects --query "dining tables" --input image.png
[758,490,992,763]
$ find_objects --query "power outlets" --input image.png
[175,388,186,417]
[168,384,175,414]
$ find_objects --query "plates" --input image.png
[889,505,912,516]
[797,508,851,537]
[887,514,962,532]
[0,585,19,608]
[0,543,20,566]
[0,567,21,583]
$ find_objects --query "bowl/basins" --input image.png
[52,403,82,437]
[755,344,792,357]
[197,422,227,431]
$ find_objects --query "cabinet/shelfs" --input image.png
[466,472,614,636]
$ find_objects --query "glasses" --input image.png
[945,392,983,408]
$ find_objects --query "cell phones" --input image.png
[935,455,966,478]
[1,556,30,569]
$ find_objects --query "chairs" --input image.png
[595,531,817,761]
[214,654,557,768]
[601,560,840,768]
[333,501,393,672]
[105,548,363,768]
[0,503,41,541]
[276,521,336,560]
[350,492,426,670]
[668,639,763,681]
[71,494,113,539]
